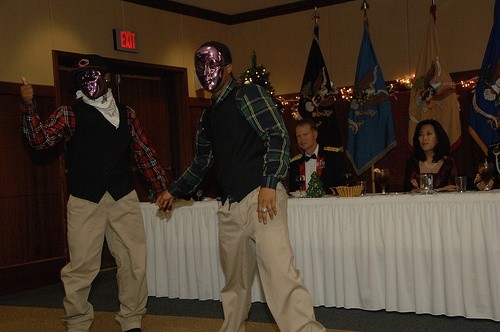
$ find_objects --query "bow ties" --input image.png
[303,153,317,162]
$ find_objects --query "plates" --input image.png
[289,192,305,196]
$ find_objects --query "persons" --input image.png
[287,118,361,195]
[19,55,171,332]
[470,126,500,191]
[155,41,327,332]
[404,119,460,192]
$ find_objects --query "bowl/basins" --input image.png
[330,187,337,195]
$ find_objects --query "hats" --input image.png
[68,55,106,74]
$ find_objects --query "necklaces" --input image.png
[422,157,442,170]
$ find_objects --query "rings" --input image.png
[261,208,268,213]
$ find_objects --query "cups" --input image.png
[357,181,366,195]
[420,175,434,195]
[455,177,467,192]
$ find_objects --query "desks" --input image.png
[138,189,500,321]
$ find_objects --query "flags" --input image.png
[465,0,500,164]
[346,12,397,176]
[297,18,341,162]
[407,1,464,160]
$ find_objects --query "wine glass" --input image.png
[376,168,390,195]
[296,175,305,198]
[479,163,494,191]
